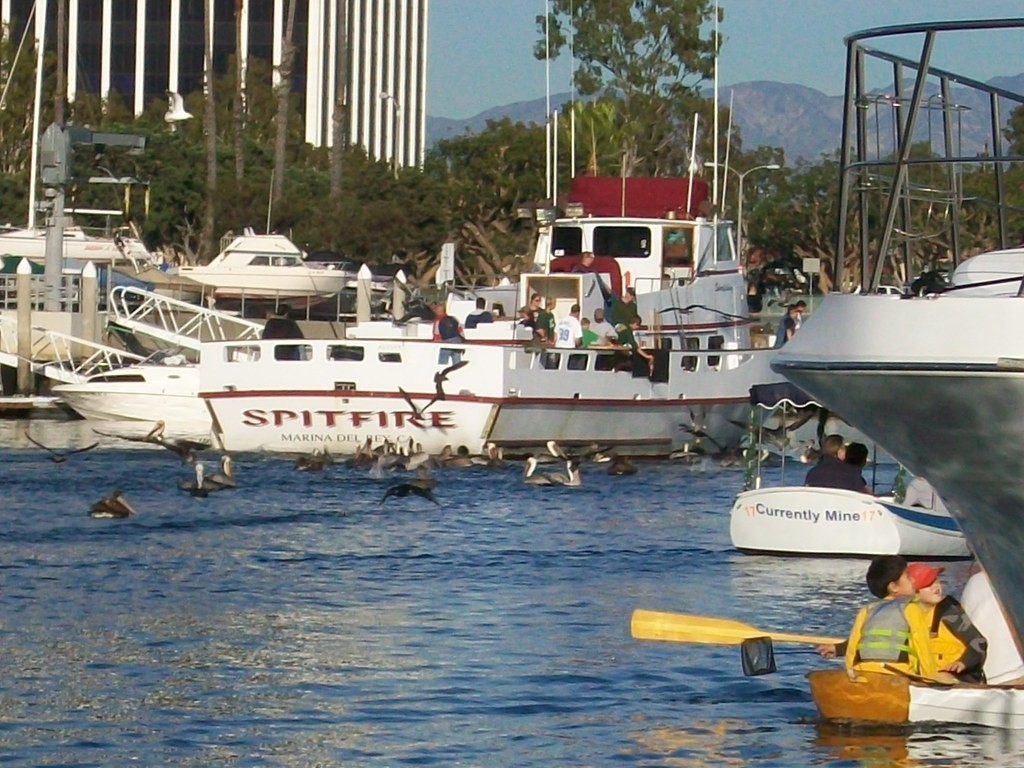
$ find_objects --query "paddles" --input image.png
[629,608,852,646]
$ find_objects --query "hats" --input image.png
[867,556,907,599]
[906,561,944,589]
[626,287,635,296]
[594,308,604,319]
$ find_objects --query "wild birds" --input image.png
[19,343,834,519]
[163,89,195,124]
[385,276,432,329]
[658,304,750,324]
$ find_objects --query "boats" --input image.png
[50,0,788,459]
[805,662,1024,731]
[769,17,1024,666]
[730,379,976,561]
[0,0,356,392]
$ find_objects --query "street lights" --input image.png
[704,161,780,264]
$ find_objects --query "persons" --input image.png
[804,434,878,496]
[261,304,307,361]
[773,300,808,349]
[817,555,988,685]
[747,281,763,322]
[611,287,638,323]
[571,251,616,307]
[174,248,190,267]
[431,294,654,365]
